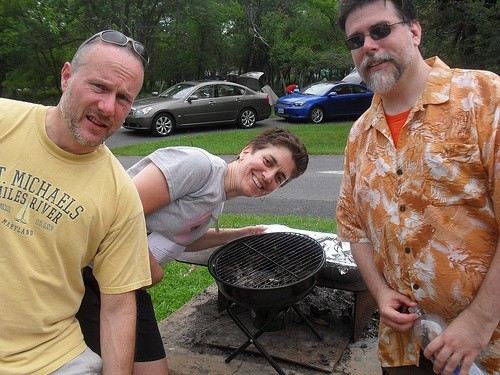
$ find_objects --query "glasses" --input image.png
[343,20,409,51]
[76,28,151,69]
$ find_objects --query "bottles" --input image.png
[407,305,491,375]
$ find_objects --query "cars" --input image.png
[274,81,375,124]
[122,71,272,137]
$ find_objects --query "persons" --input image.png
[0,30,153,375]
[335,0,500,375]
[76,127,309,375]
[284,84,299,96]
